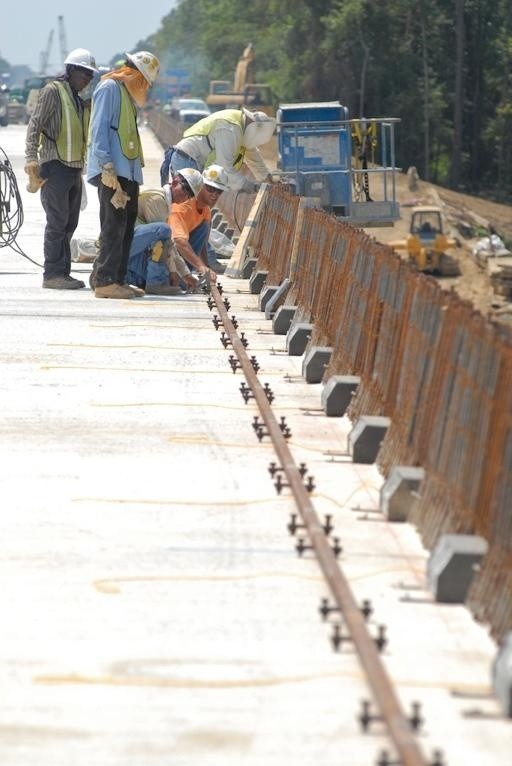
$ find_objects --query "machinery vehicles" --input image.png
[384,205,466,279]
[206,37,278,133]
[0,69,59,129]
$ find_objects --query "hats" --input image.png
[243,106,271,122]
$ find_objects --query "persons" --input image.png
[23,47,100,291]
[87,50,161,298]
[127,167,202,296]
[168,105,278,274]
[168,163,231,291]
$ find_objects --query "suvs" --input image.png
[167,93,212,126]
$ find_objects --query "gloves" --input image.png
[111,182,131,210]
[100,164,118,190]
[24,163,48,193]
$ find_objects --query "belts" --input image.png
[173,148,194,161]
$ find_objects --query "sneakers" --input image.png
[95,282,145,299]
[146,284,182,295]
[42,275,85,289]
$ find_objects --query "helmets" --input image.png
[199,164,229,192]
[63,48,100,75]
[177,168,204,201]
[126,51,159,87]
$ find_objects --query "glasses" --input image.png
[180,180,194,197]
[206,185,224,195]
